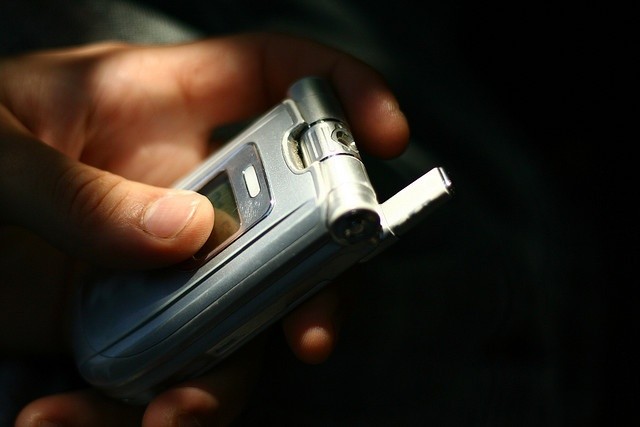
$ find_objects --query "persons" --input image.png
[1,40,412,426]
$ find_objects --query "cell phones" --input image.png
[59,78,457,399]
